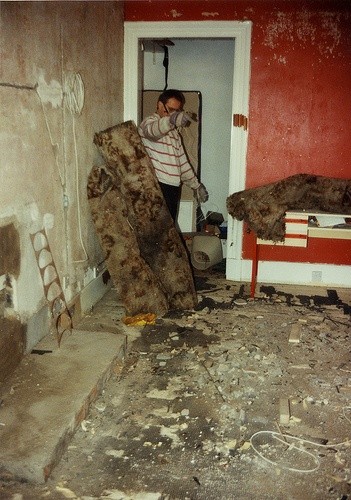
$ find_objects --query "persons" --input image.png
[137,89,209,223]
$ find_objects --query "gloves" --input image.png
[195,185,208,203]
[170,112,187,127]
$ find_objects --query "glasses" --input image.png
[164,104,182,114]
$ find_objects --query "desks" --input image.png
[250,211,351,298]
